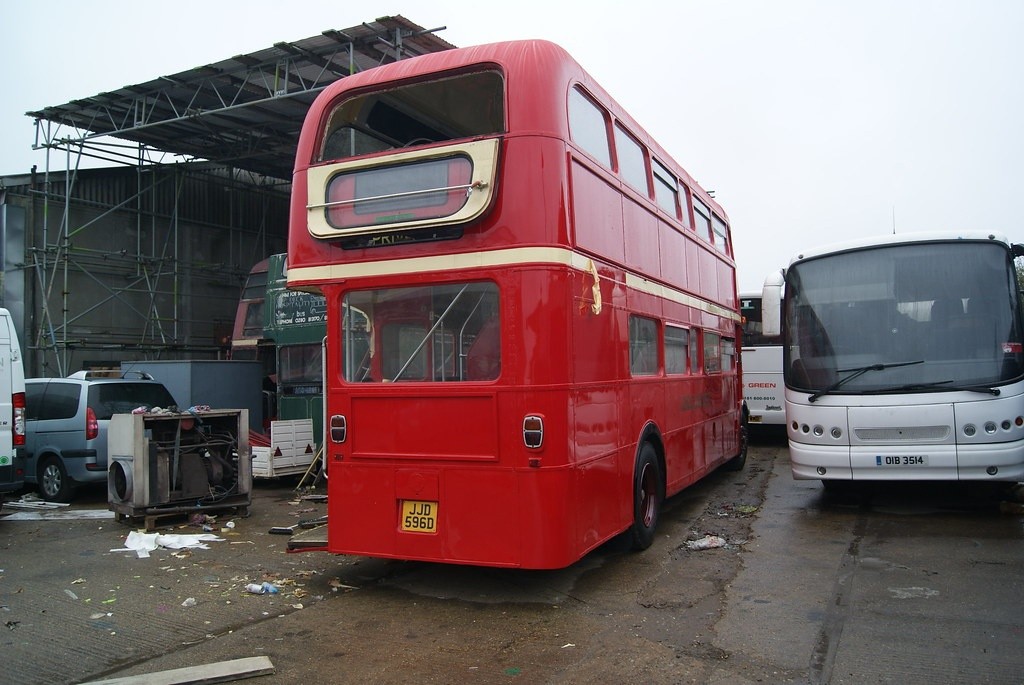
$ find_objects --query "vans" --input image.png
[0,308,25,497]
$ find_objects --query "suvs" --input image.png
[26,368,179,505]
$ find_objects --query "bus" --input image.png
[284,41,749,573]
[733,290,786,425]
[229,252,472,469]
[762,227,1024,492]
